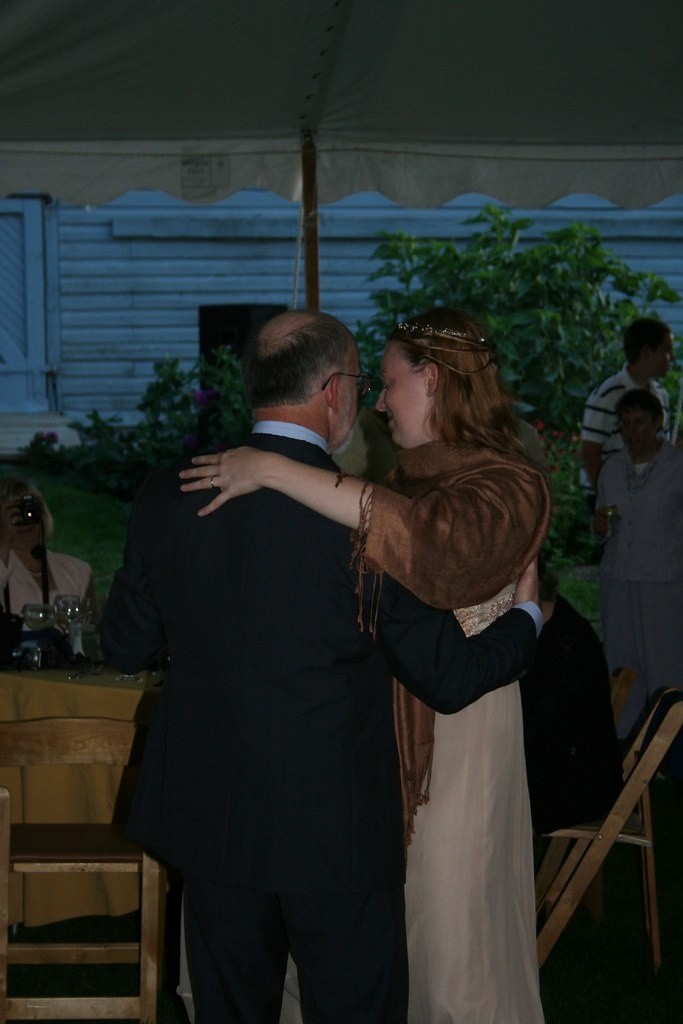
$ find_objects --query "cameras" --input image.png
[9,494,44,525]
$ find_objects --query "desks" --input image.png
[0,620,178,926]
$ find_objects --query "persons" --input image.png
[92,310,544,1023]
[518,554,626,883]
[0,476,100,655]
[178,308,552,1024]
[580,317,683,781]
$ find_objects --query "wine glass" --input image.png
[22,596,106,631]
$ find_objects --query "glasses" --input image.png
[619,417,658,428]
[321,372,371,398]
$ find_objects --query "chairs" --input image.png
[0,718,170,1024]
[534,668,683,974]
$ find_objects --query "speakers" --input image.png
[199,302,289,393]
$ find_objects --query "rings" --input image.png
[11,520,16,526]
[210,476,217,488]
